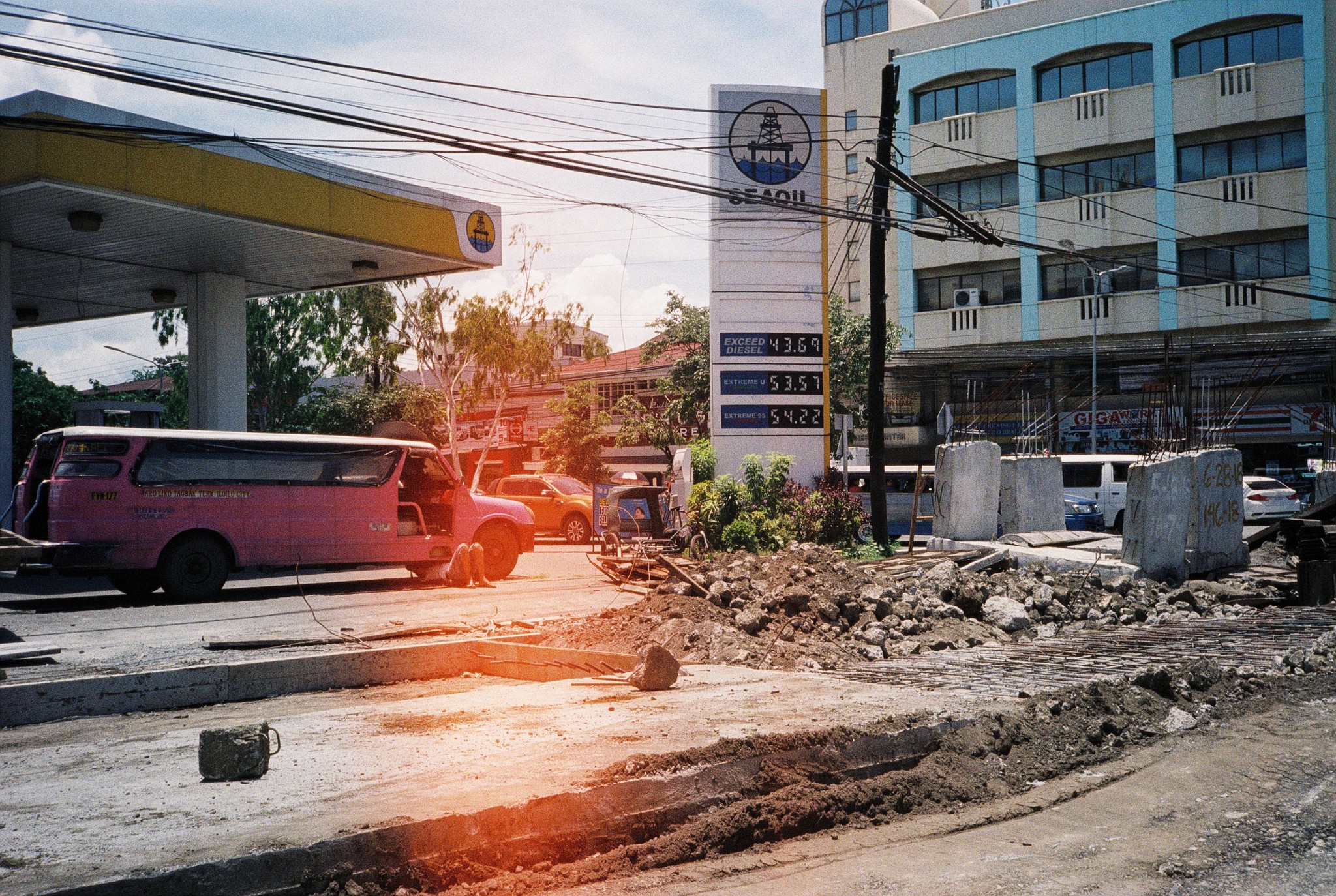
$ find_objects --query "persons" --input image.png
[633,507,646,520]
[405,542,496,589]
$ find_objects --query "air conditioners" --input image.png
[954,287,980,308]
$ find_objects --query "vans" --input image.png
[14,425,536,599]
[833,464,1107,543]
[1049,453,1153,535]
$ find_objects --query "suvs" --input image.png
[480,473,593,543]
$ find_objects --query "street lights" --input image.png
[104,345,188,399]
[1059,239,1137,455]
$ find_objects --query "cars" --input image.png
[1241,474,1301,524]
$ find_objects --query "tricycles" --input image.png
[600,486,713,562]
